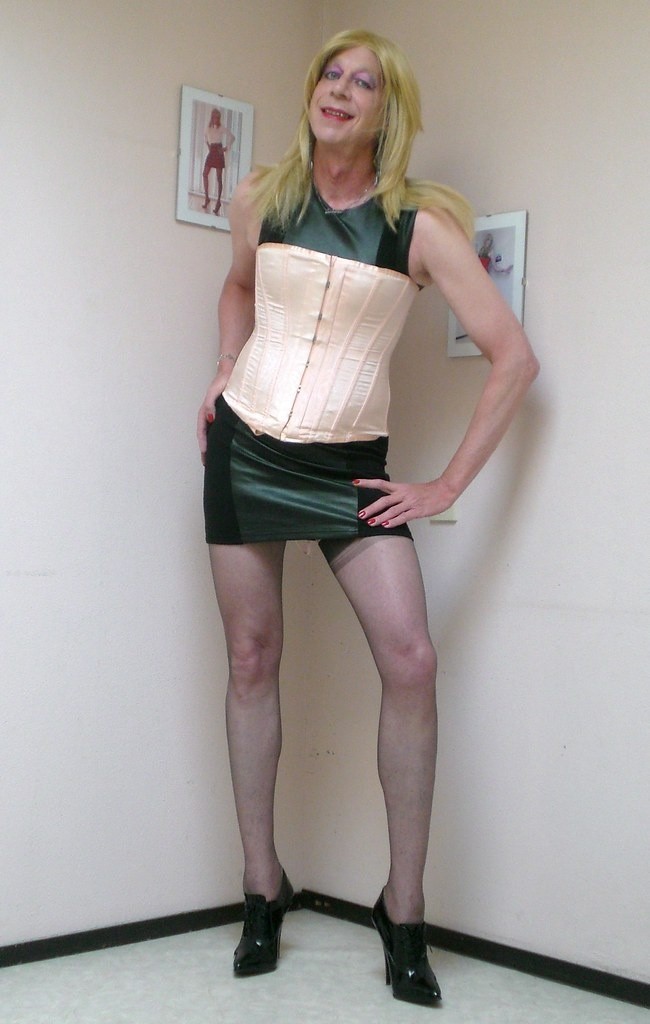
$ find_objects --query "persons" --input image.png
[199,30,539,1002]
[478,234,501,275]
[201,109,235,213]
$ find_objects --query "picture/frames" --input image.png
[445,209,528,357]
[177,85,255,233]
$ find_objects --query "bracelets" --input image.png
[217,354,237,367]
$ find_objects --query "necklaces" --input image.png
[311,161,378,213]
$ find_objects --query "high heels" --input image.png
[234,869,294,975]
[213,204,221,213]
[203,199,210,208]
[371,886,441,1004]
